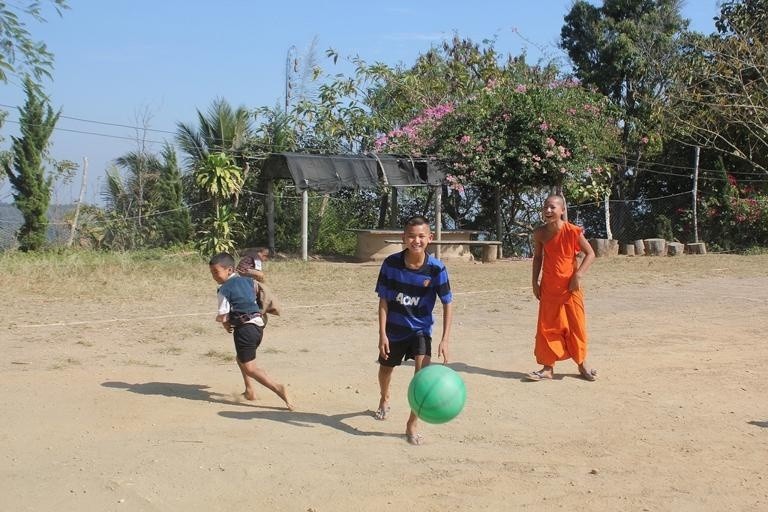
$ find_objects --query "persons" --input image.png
[375,216,454,448]
[525,194,600,382]
[210,248,297,411]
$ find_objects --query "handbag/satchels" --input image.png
[230,316,244,326]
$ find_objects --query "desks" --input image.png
[348,229,489,259]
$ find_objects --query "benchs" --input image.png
[386,240,502,263]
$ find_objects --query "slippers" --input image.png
[375,406,391,420]
[406,432,424,445]
[580,369,598,381]
[525,370,556,380]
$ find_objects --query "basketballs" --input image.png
[408,364,466,423]
